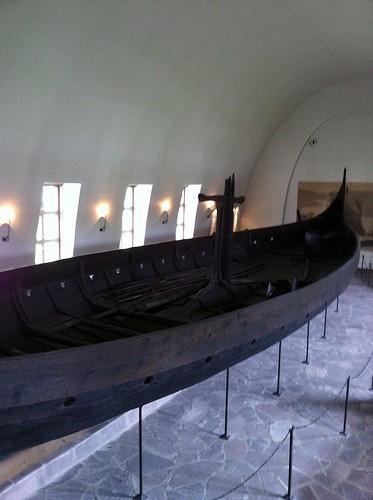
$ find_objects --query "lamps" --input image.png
[0,203,15,241]
[159,197,170,224]
[206,201,216,218]
[95,201,110,233]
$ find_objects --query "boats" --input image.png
[0,166,360,459]
